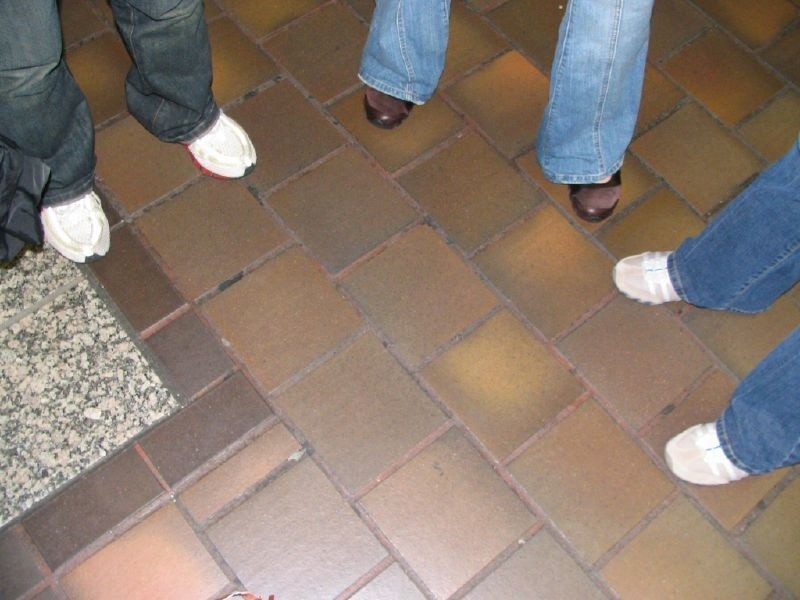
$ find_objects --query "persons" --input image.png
[0,0,257,264]
[612,136,800,486]
[357,0,655,223]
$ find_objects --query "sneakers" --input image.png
[40,191,110,263]
[664,421,749,486]
[189,109,258,179]
[612,250,682,304]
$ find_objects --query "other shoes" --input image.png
[364,86,415,128]
[570,170,623,223]
